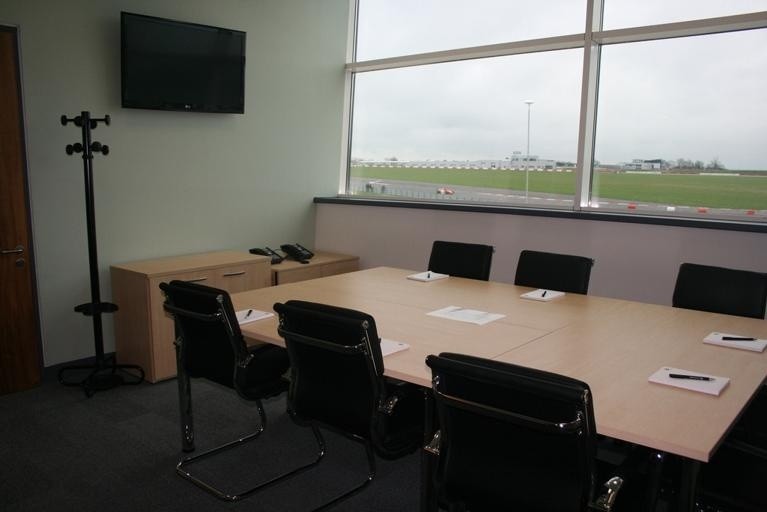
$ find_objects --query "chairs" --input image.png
[425,242,496,283]
[413,349,637,512]
[670,259,765,320]
[240,298,429,510]
[157,277,328,509]
[513,247,596,296]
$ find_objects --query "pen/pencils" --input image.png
[428,272,431,278]
[721,335,758,341]
[541,291,548,297]
[243,309,254,319]
[669,373,711,380]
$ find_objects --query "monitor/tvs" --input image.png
[120,10,246,113]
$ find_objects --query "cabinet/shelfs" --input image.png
[109,248,360,385]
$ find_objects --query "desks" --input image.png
[204,266,765,469]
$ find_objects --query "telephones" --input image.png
[279,241,315,264]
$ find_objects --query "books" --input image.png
[520,289,566,301]
[648,367,729,396]
[235,309,273,324]
[406,271,449,282]
[703,331,767,353]
[380,340,410,357]
